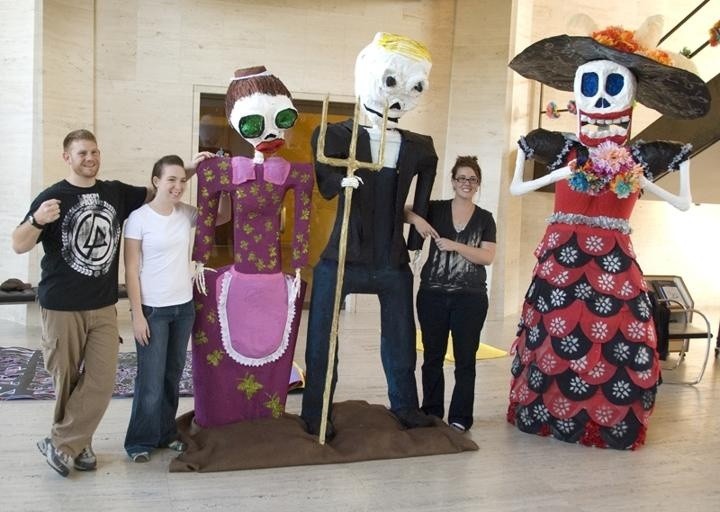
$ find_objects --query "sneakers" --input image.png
[74,447,97,471]
[450,422,466,434]
[37,435,73,477]
[131,452,149,463]
[168,440,186,452]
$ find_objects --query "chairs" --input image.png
[646,290,713,385]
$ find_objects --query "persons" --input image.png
[299,31,440,440]
[188,65,314,428]
[11,128,222,478]
[404,155,497,436]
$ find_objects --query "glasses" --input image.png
[453,177,478,183]
